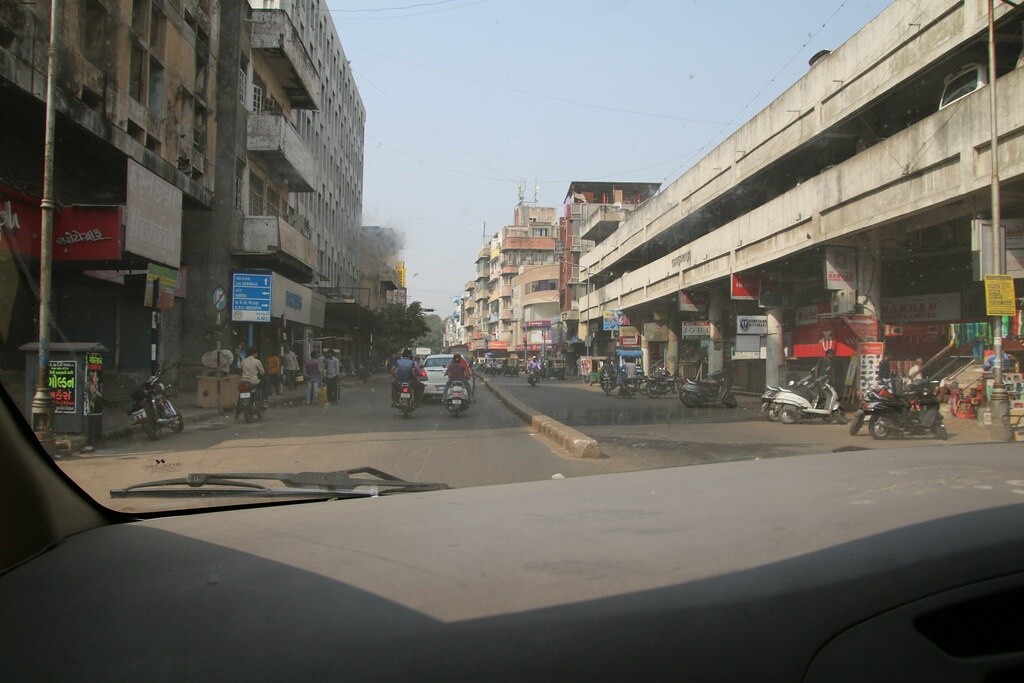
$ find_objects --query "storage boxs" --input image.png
[971,398,978,404]
[977,384,984,391]
[1002,372,1024,410]
[977,393,983,400]
[983,412,992,425]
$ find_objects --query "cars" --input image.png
[420,353,475,400]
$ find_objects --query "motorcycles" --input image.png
[442,363,472,419]
[575,348,739,409]
[395,371,428,419]
[128,364,185,441]
[470,353,568,387]
[238,373,265,424]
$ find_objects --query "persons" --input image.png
[820,348,833,385]
[239,340,247,359]
[908,358,923,380]
[322,349,340,405]
[234,348,268,422]
[284,346,299,391]
[265,351,281,396]
[484,359,497,364]
[878,351,893,386]
[601,357,638,390]
[390,349,425,409]
[442,352,476,404]
[307,351,321,405]
[527,356,541,370]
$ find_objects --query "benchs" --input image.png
[1001,414,1024,442]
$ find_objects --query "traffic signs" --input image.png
[233,273,272,287]
[232,286,272,300]
[231,298,271,322]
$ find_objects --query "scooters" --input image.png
[759,364,949,442]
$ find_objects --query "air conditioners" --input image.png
[831,289,857,319]
